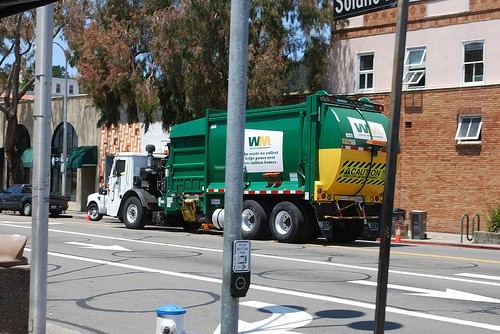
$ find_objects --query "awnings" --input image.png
[67,145,97,169]
[18,147,58,167]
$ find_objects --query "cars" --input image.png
[86,187,108,222]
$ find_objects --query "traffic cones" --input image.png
[393,226,402,243]
[86,209,91,221]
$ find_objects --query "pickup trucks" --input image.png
[0,183,69,218]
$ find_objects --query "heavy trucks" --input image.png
[98,90,400,244]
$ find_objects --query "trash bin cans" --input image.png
[392,207,406,237]
[409,210,428,239]
